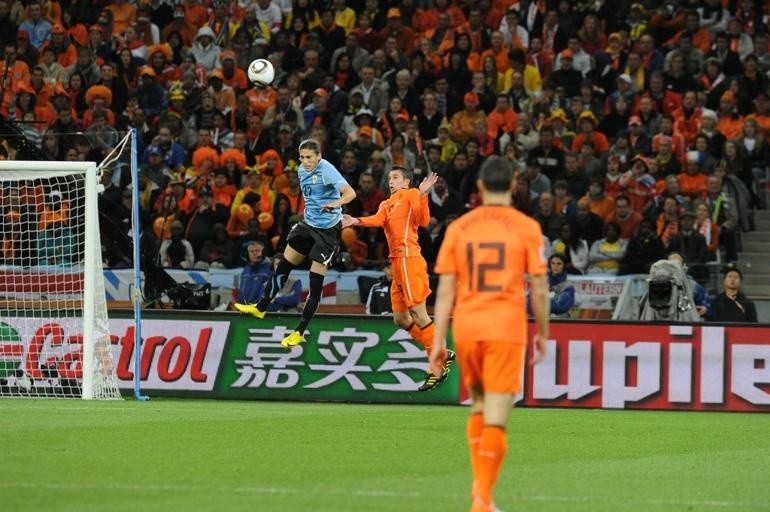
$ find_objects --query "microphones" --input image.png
[257,255,264,258]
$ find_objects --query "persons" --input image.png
[342,164,456,392]
[235,139,357,347]
[430,154,549,512]
[0,0,770,321]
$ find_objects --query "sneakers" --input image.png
[418,349,456,392]
[280,331,305,347]
[234,304,267,319]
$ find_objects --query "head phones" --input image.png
[665,251,689,275]
[547,254,569,270]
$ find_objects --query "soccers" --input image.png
[249,58,274,87]
